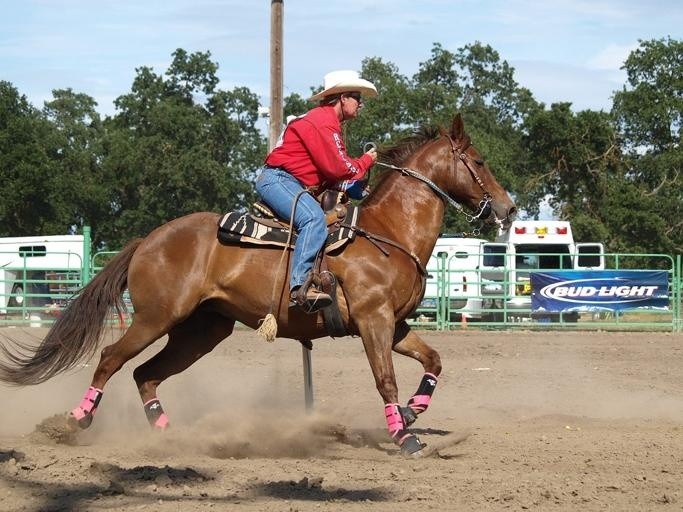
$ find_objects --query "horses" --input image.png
[0,112,519,461]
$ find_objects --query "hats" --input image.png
[308,69,379,102]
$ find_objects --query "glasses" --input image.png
[342,94,361,101]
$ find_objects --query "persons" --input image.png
[255,70,380,312]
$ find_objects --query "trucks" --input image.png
[477,219,605,321]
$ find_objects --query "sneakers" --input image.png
[288,286,332,314]
